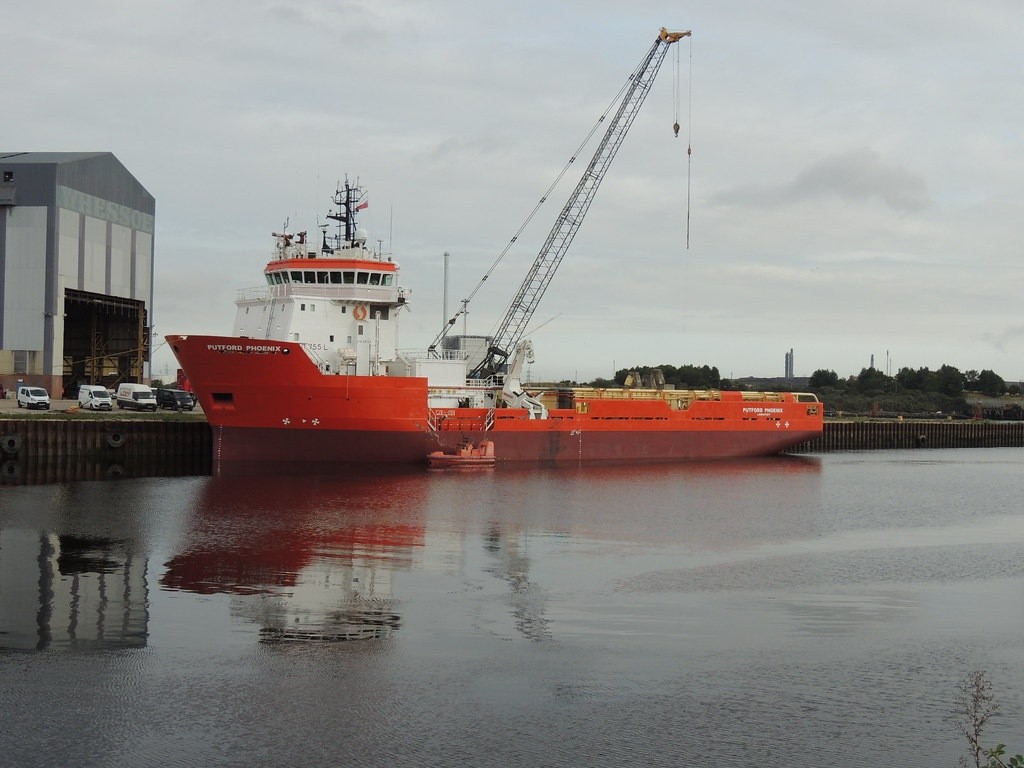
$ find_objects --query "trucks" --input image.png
[117,383,158,411]
[151,387,197,411]
[79,385,113,411]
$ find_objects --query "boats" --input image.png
[164,27,825,476]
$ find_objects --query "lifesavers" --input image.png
[1,459,22,479]
[107,463,128,479]
[918,435,927,446]
[105,427,128,448]
[0,432,23,454]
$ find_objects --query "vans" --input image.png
[18,387,50,410]
